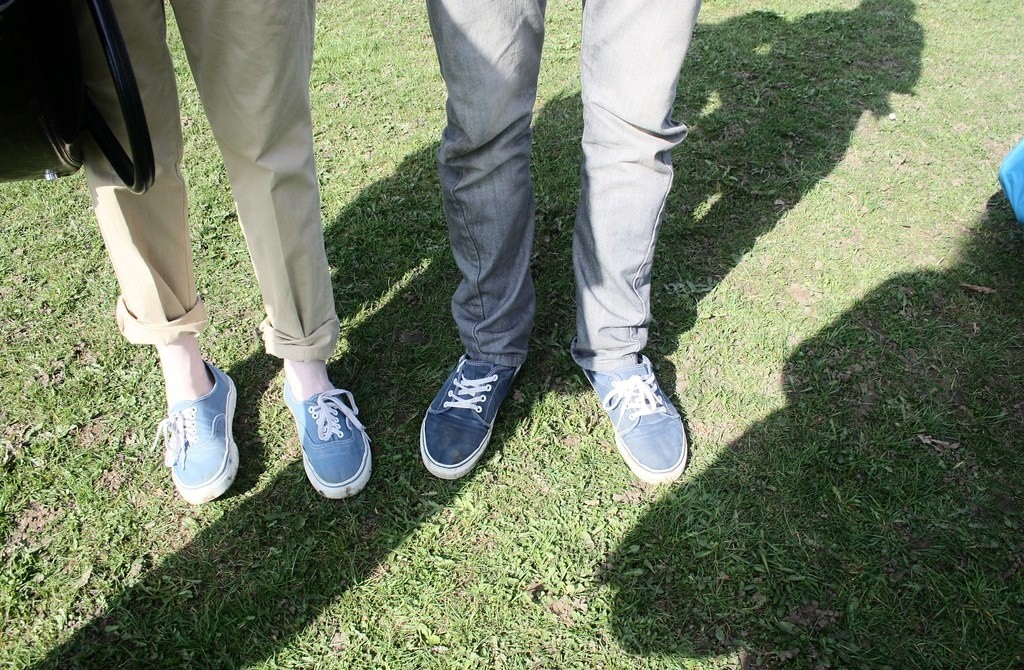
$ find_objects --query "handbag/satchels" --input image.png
[0,0,159,196]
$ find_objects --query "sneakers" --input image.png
[418,351,521,480]
[283,379,372,499]
[582,354,688,485]
[149,358,239,507]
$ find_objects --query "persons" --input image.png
[82,0,374,504]
[419,0,691,482]
